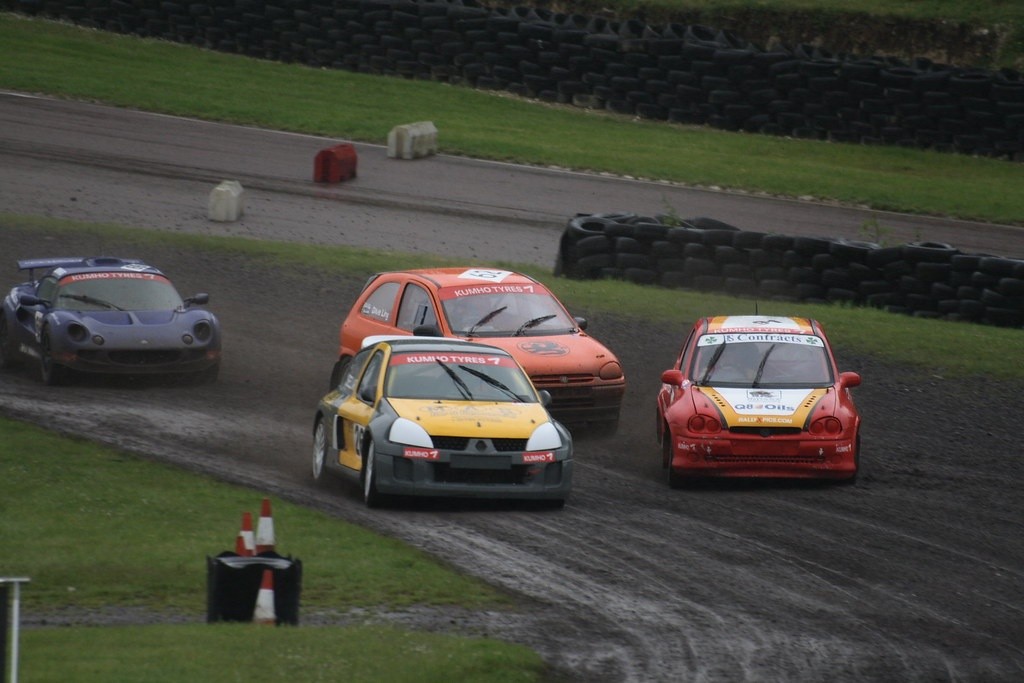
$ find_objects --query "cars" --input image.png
[0,257,222,384]
[329,267,625,436]
[310,336,575,508]
[656,315,861,483]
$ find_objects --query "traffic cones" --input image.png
[235,512,258,558]
[251,569,279,627]
[256,499,276,557]
